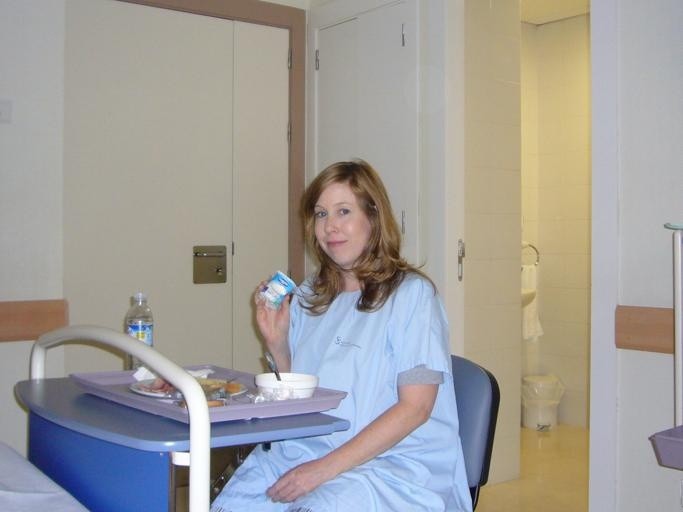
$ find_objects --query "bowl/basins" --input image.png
[253,371,321,401]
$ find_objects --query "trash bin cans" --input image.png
[522,375,564,430]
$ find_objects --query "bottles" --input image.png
[123,291,153,370]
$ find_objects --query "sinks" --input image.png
[520,289,540,315]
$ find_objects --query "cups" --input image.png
[257,269,297,311]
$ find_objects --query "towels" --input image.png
[521,264,544,339]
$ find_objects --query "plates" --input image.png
[67,362,350,426]
[127,376,249,400]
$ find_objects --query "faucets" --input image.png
[520,241,540,276]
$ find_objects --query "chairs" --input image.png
[448,353,500,512]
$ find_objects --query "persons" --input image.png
[203,159,474,512]
